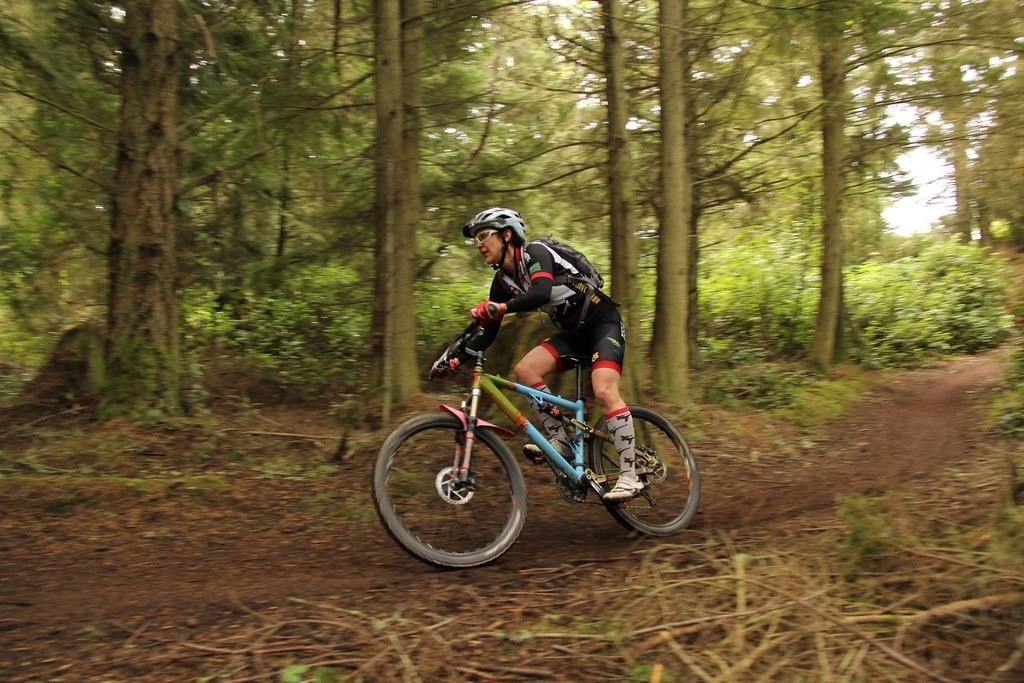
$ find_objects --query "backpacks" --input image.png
[523,238,604,289]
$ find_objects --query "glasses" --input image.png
[474,230,498,245]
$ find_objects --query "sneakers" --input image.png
[603,475,644,503]
[523,438,575,462]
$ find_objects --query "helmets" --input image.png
[462,207,526,244]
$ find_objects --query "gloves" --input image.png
[468,300,502,322]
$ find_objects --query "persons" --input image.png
[430,207,652,501]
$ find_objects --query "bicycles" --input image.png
[371,306,703,570]
